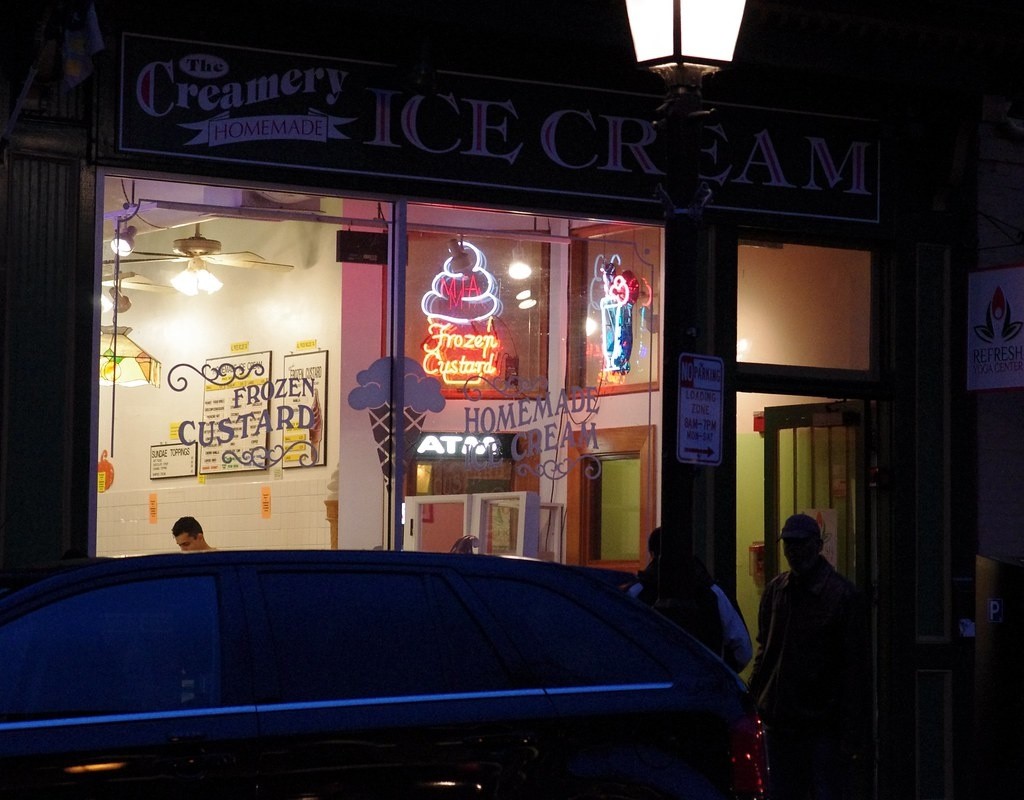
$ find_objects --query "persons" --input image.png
[746,514,866,800]
[627,525,754,674]
[171,516,218,551]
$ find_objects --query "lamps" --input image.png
[109,287,132,313]
[623,0,749,117]
[170,257,223,296]
[110,226,137,257]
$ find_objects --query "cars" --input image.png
[1,549,778,800]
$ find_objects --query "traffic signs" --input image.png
[676,351,725,467]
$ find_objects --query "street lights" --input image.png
[622,0,749,571]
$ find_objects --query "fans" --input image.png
[102,223,296,296]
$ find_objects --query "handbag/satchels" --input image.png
[711,584,754,672]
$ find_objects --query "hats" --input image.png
[782,514,821,539]
